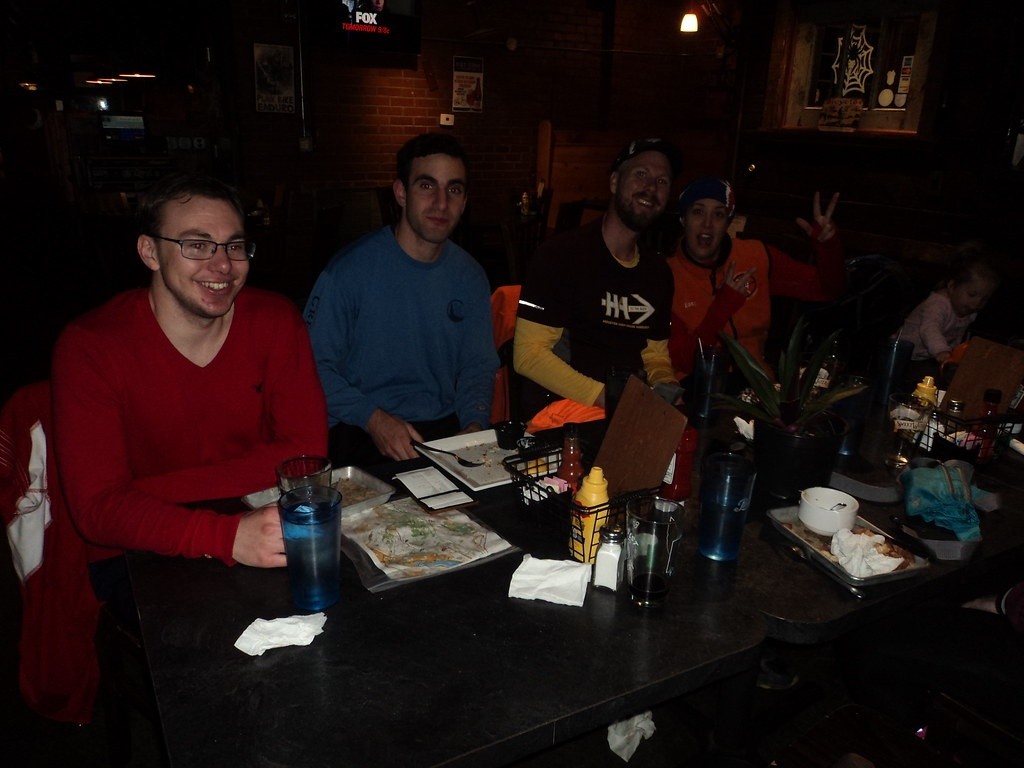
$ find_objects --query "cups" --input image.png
[493,421,528,450]
[874,337,915,405]
[277,484,343,610]
[697,452,757,561]
[276,454,331,496]
[624,495,686,608]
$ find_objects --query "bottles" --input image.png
[946,399,965,434]
[264,206,271,226]
[969,389,1004,463]
[593,523,626,593]
[561,423,582,507]
[257,198,263,208]
[813,341,840,389]
[569,467,611,565]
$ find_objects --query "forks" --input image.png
[410,439,487,467]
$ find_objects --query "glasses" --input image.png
[150,233,257,261]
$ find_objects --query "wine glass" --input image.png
[883,393,933,471]
[697,336,732,418]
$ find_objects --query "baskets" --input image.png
[891,387,1024,463]
[501,437,664,565]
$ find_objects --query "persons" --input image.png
[347,0,399,48]
[666,176,849,385]
[512,139,680,434]
[886,245,997,388]
[302,129,501,468]
[51,172,327,628]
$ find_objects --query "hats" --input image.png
[611,134,674,178]
[675,174,736,219]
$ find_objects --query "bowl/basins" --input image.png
[798,487,860,537]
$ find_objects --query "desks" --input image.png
[123,419,769,768]
[522,374,1024,756]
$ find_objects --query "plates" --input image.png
[414,428,561,492]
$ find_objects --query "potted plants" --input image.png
[710,314,871,512]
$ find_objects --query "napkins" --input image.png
[506,553,592,607]
[232,611,328,661]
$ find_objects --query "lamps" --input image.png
[679,0,698,33]
